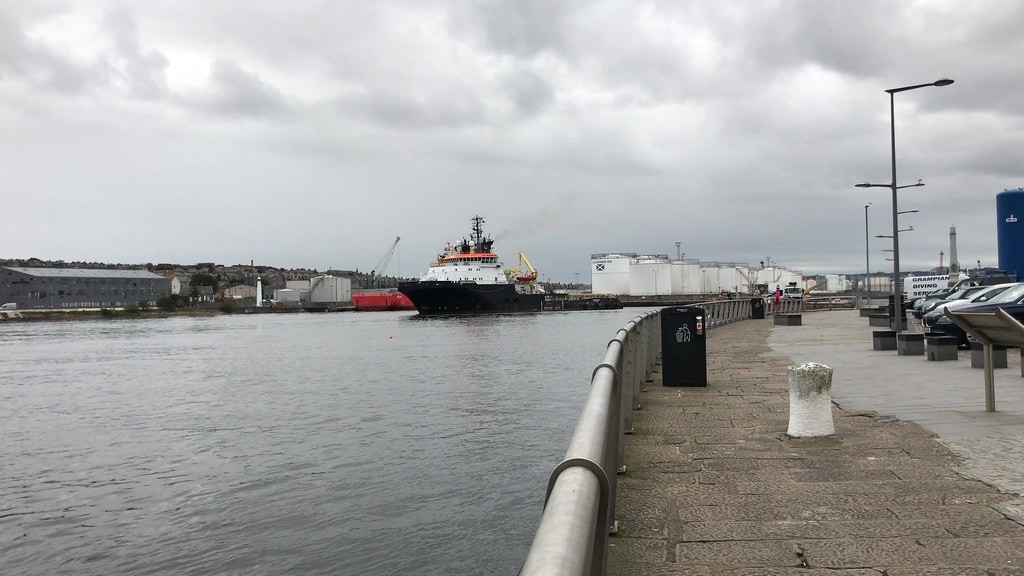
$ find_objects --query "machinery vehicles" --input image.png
[780,278,828,301]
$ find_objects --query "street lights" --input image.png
[856,77,959,328]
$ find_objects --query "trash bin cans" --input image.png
[751,297,764,319]
[661,307,707,387]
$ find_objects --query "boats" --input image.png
[350,292,417,312]
[396,212,548,316]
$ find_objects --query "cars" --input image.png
[903,275,1024,350]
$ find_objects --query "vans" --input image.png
[1,303,17,310]
